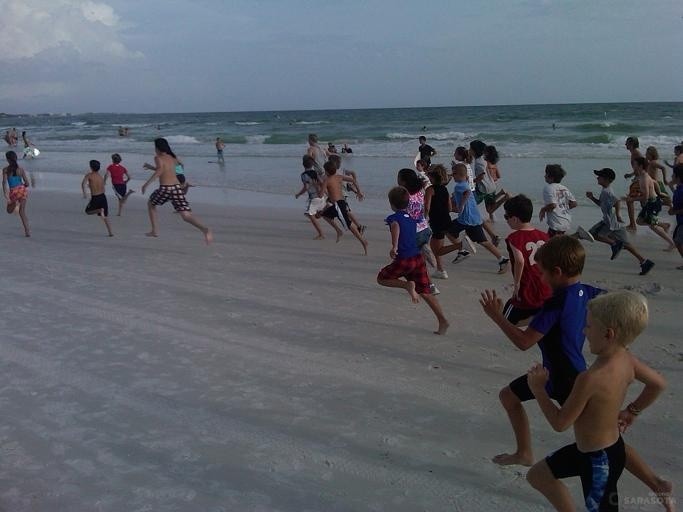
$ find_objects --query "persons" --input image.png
[118,126,123,137]
[1,149,32,238]
[5,131,10,147]
[173,163,196,196]
[104,153,136,217]
[79,159,114,237]
[294,133,369,257]
[215,137,225,162]
[10,127,18,146]
[375,135,682,511]
[22,131,34,161]
[140,136,211,245]
[124,128,128,136]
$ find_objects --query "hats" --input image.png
[593,168,616,180]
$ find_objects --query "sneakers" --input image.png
[576,224,595,244]
[492,235,502,249]
[460,235,477,256]
[429,282,440,296]
[610,240,624,260]
[359,224,369,241]
[430,270,449,280]
[451,249,472,264]
[497,256,511,274]
[639,258,655,275]
